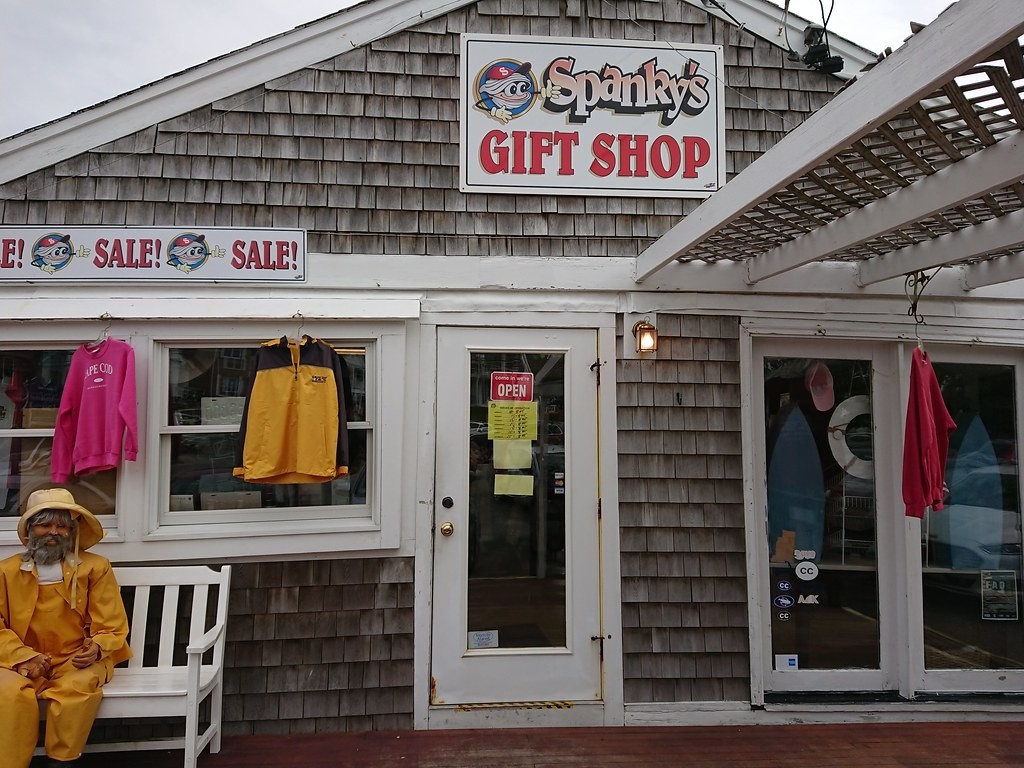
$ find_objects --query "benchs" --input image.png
[20,563,231,768]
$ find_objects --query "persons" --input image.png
[0,488,133,768]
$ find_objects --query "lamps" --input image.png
[632,316,659,353]
[801,21,844,76]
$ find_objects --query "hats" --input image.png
[804,361,835,412]
[17,488,104,552]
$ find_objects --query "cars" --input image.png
[921,461,1022,600]
[470,401,565,495]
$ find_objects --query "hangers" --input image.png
[85,311,112,350]
[915,321,927,356]
[284,312,308,348]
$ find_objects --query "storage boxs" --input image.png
[199,471,262,512]
